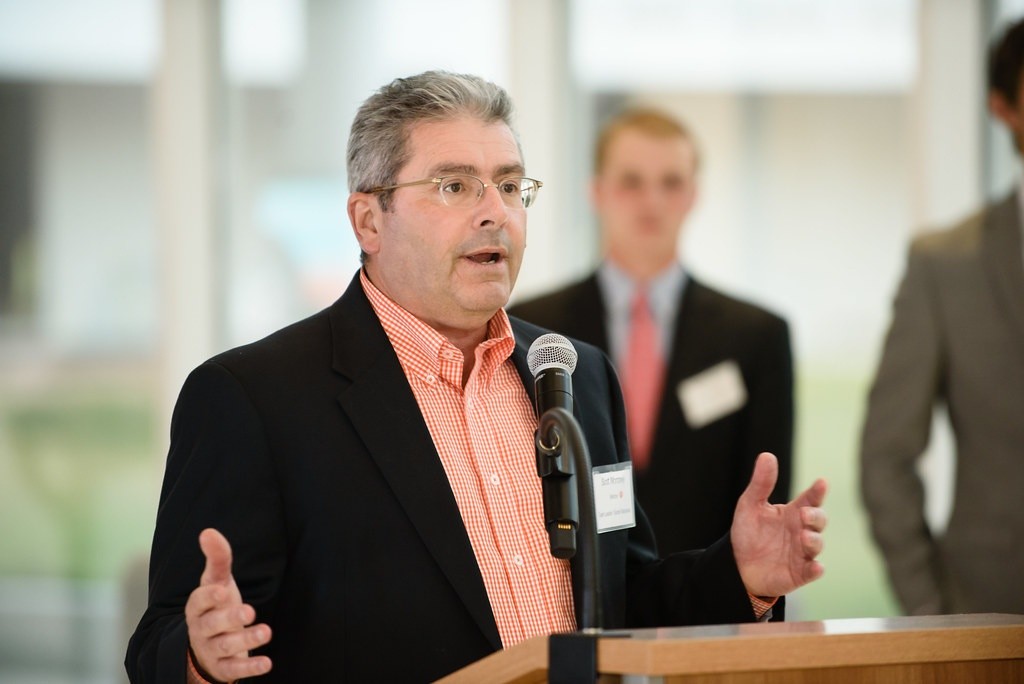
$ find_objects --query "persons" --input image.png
[859,17,1024,617]
[124,69,827,684]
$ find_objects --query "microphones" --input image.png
[528,333,578,560]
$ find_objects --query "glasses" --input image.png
[364,174,544,211]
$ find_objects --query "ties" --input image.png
[616,279,665,473]
